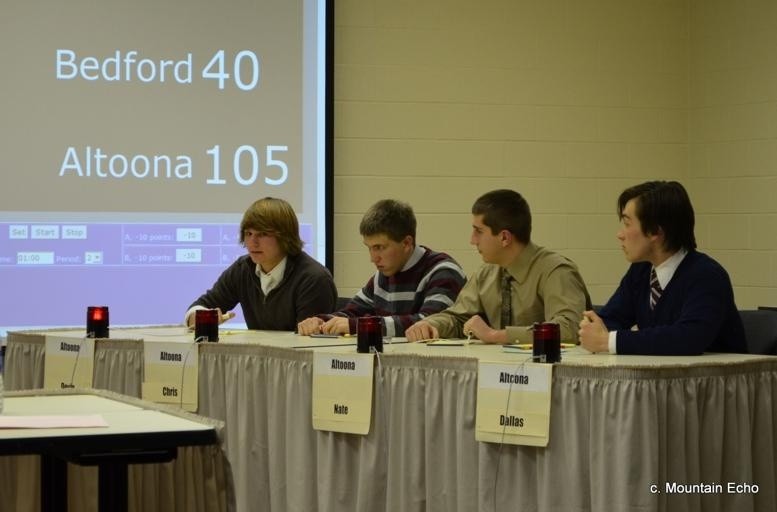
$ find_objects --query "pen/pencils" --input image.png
[188,314,231,330]
[583,315,596,354]
[340,333,351,337]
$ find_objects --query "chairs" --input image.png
[737,309,776,354]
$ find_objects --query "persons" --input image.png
[405,189,592,345]
[184,198,338,330]
[577,180,750,354]
[296,199,469,339]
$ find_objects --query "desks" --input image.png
[0,390,214,512]
[0,325,777,512]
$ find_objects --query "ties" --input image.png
[499,274,511,329]
[648,269,662,309]
